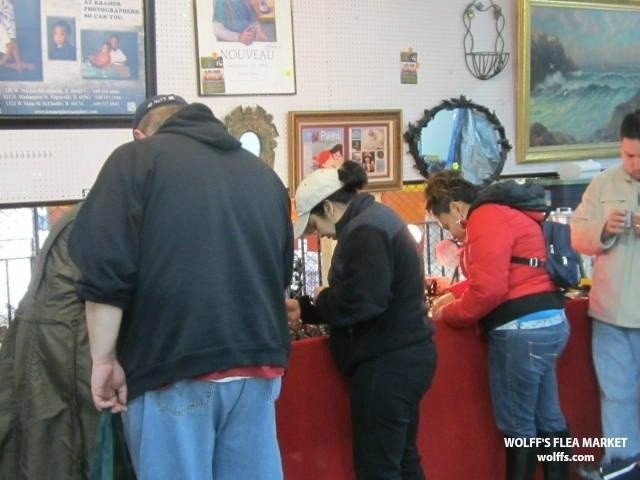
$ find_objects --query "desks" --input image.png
[277,283,605,479]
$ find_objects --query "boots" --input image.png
[505,438,538,479]
[539,428,571,480]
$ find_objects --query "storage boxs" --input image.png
[544,208,595,288]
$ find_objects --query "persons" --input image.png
[70,92,295,480]
[81,33,128,80]
[285,161,437,480]
[0,24,13,74]
[567,109,639,479]
[211,0,267,46]
[48,20,73,58]
[316,143,346,168]
[0,195,134,478]
[351,129,385,174]
[0,0,36,74]
[110,34,127,72]
[422,169,572,479]
[206,51,220,80]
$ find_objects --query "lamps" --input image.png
[409,224,428,245]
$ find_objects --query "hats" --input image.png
[293,169,345,237]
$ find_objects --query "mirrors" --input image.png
[224,104,281,170]
[404,96,514,187]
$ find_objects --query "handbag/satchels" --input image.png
[541,220,583,290]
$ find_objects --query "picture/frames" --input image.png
[289,108,405,194]
[193,0,300,98]
[513,1,640,163]
[0,2,158,132]
